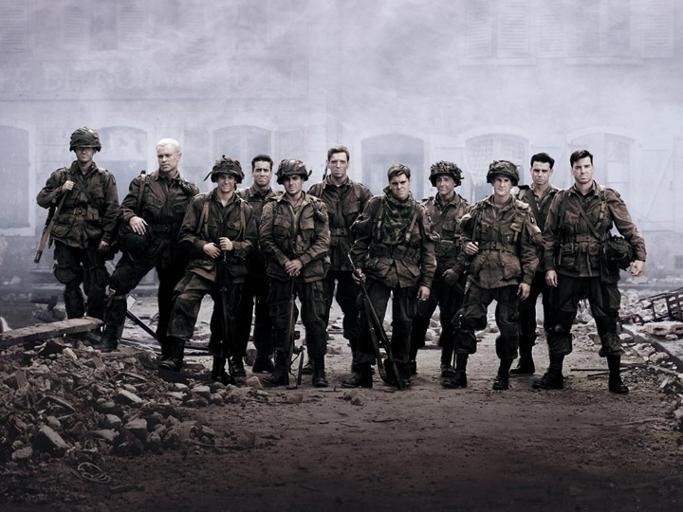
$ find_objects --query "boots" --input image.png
[212,353,236,384]
[408,345,417,376]
[101,323,123,351]
[309,354,327,386]
[262,351,291,385]
[607,355,629,394]
[229,353,246,384]
[441,347,455,376]
[342,362,374,388]
[385,350,407,381]
[492,360,512,390]
[441,359,467,388]
[253,344,274,372]
[160,342,184,372]
[532,353,564,389]
[510,347,535,374]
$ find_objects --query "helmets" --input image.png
[429,161,462,187]
[487,160,519,186]
[70,127,101,152]
[608,235,635,269]
[212,161,244,183]
[277,160,308,184]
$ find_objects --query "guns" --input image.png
[282,275,303,385]
[33,207,55,263]
[218,251,234,385]
[341,240,406,391]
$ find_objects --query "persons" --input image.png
[36,127,121,336]
[509,152,564,375]
[338,164,437,389]
[260,159,331,387]
[441,160,543,390]
[409,160,471,377]
[302,146,376,375]
[98,138,199,353]
[160,155,259,384]
[533,150,647,393]
[227,155,283,377]
